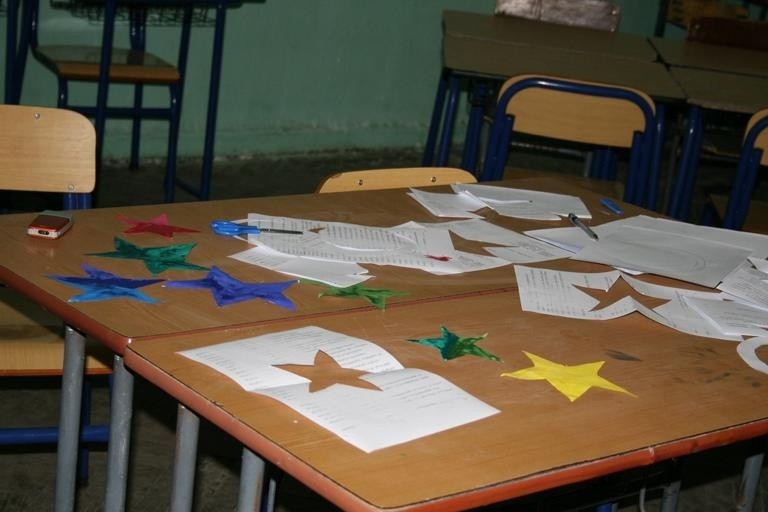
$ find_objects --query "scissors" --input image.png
[211,219,304,237]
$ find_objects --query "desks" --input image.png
[2,179,680,511]
[124,258,766,508]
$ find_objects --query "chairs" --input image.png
[2,0,182,207]
[0,102,116,487]
[424,0,768,234]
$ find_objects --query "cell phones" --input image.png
[27,213,73,240]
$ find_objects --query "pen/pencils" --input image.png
[569,213,599,240]
